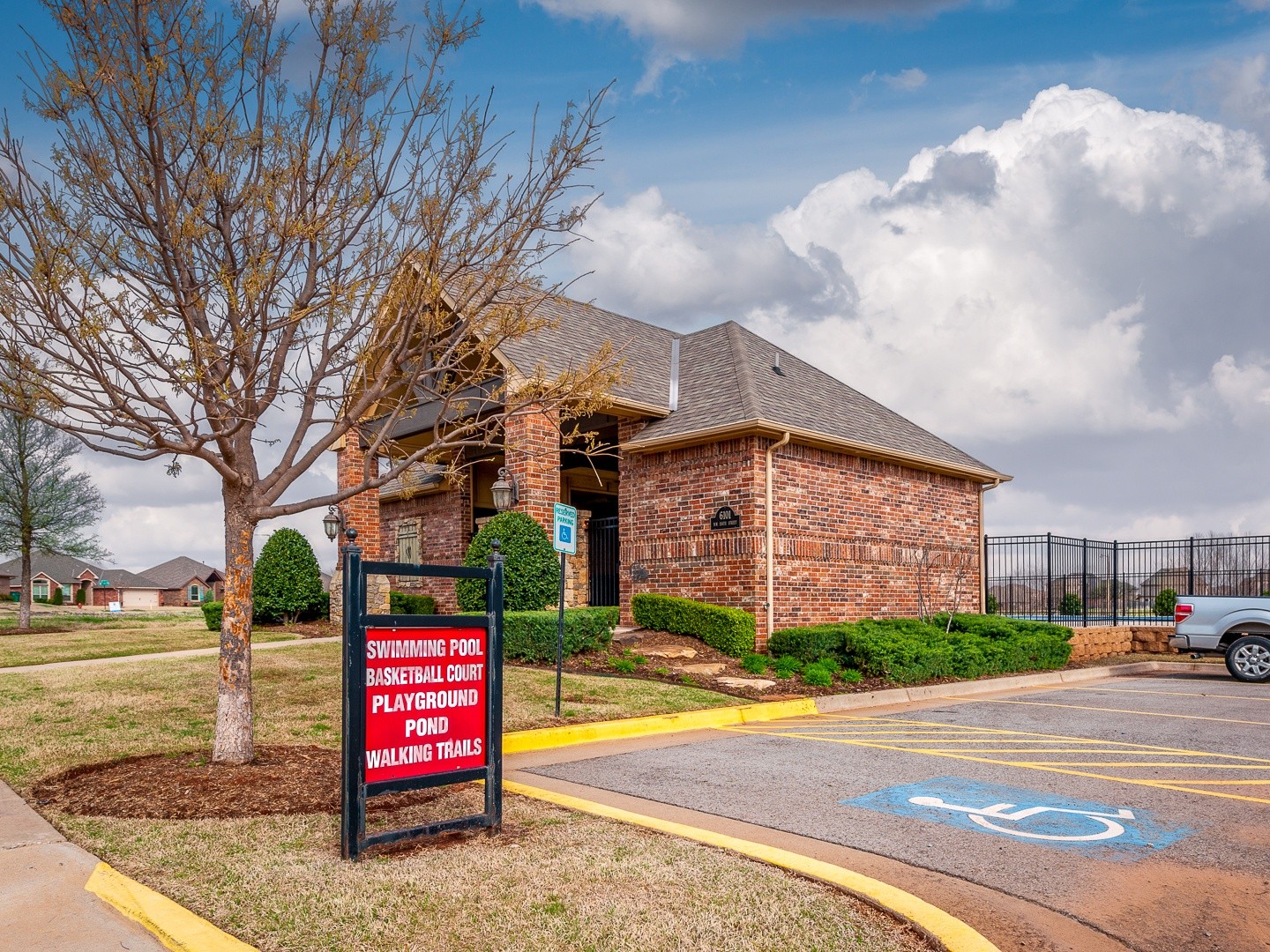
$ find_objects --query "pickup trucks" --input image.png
[1171,595,1270,683]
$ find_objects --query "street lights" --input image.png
[1101,554,1111,615]
[99,580,110,612]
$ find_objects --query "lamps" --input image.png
[322,502,348,543]
[491,467,520,514]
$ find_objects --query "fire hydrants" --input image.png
[78,602,82,609]
